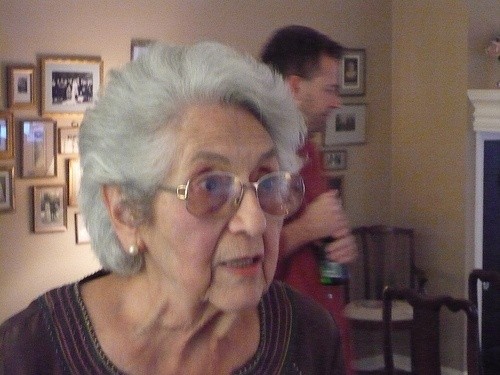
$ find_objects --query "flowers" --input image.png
[485,37,500,63]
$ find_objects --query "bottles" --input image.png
[318,176,350,285]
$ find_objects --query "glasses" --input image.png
[156,170,305,223]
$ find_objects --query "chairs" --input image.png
[338,225,500,375]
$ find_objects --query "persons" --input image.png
[0,43,346,375]
[260,25,358,375]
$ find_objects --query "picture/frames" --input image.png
[40,56,103,116]
[130,39,154,61]
[20,120,58,178]
[0,167,14,213]
[0,111,14,159]
[321,150,347,171]
[32,185,67,234]
[340,49,366,97]
[324,175,345,206]
[58,126,80,154]
[75,214,90,244]
[323,104,369,146]
[7,65,34,109]
